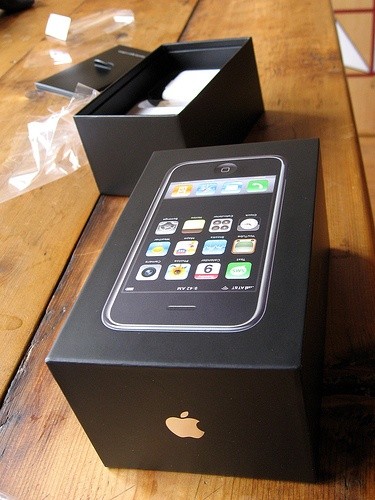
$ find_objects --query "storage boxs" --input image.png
[46,138,331,484]
[75,37,263,197]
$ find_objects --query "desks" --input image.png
[1,0,375,500]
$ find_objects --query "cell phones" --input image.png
[101,149,287,334]
